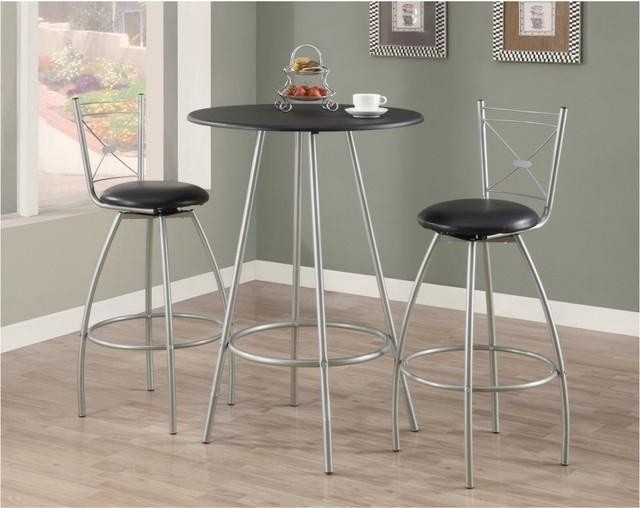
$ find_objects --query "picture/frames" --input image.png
[368,1,447,59]
[492,1,581,65]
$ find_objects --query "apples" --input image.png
[285,85,327,96]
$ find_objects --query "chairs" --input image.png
[71,92,235,436]
[392,98,570,491]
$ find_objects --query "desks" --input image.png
[188,103,424,474]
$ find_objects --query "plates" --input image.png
[346,107,387,120]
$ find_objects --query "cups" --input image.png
[354,93,387,111]
